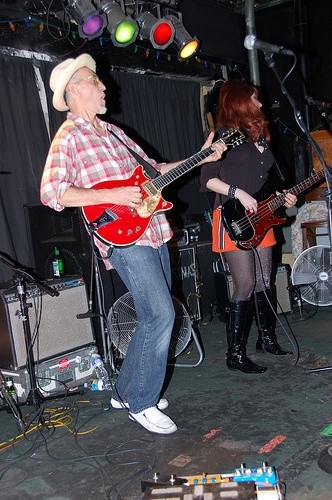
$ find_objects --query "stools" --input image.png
[301,221,328,265]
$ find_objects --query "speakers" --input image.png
[0,273,97,372]
[214,263,293,323]
[25,202,87,282]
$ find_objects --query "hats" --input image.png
[49,53,97,112]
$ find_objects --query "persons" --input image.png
[199,79,298,374]
[39,53,228,436]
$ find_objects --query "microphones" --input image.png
[286,284,308,290]
[76,313,101,320]
[244,35,294,55]
[303,96,332,108]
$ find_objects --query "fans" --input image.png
[291,245,332,306]
[108,291,204,374]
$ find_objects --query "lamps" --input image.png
[55,0,201,62]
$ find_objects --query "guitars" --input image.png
[81,128,246,249]
[222,169,327,249]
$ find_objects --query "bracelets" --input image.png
[228,185,237,198]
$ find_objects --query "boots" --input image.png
[255,285,293,356]
[226,298,268,373]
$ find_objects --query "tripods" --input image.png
[0,251,87,434]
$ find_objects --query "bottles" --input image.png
[4,377,19,413]
[84,378,115,392]
[53,246,64,279]
[90,351,109,378]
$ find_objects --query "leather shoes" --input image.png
[128,406,178,435]
[110,397,169,410]
